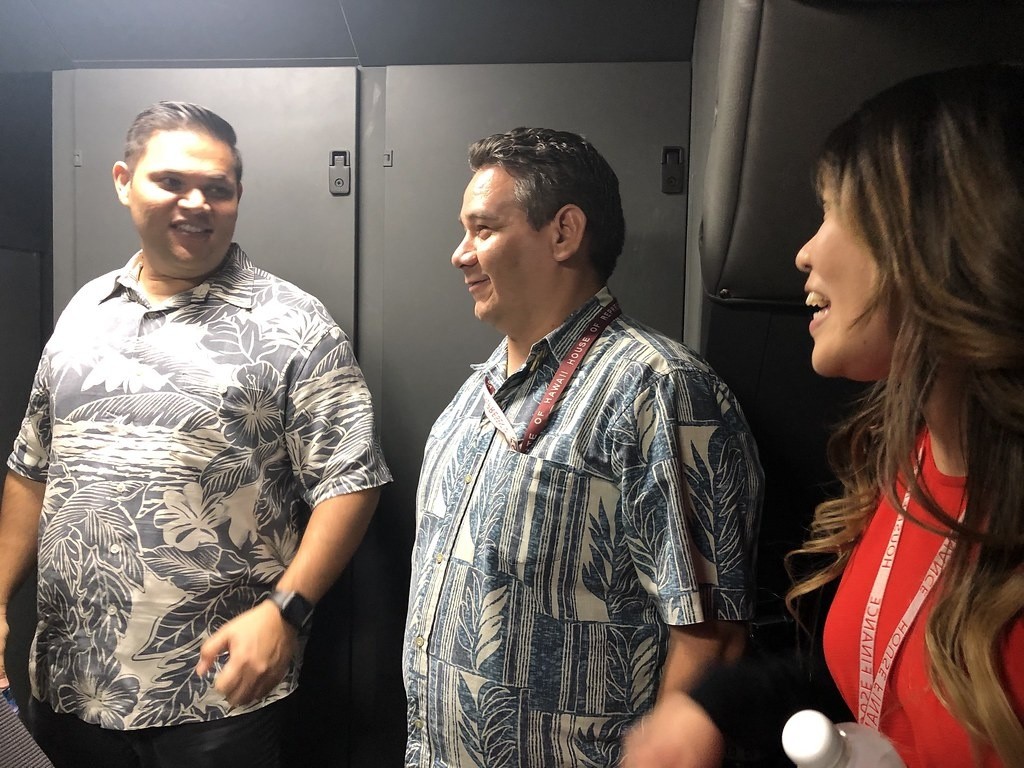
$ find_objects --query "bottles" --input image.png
[781,709,907,768]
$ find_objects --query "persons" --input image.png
[775,62,1024,768]
[0,100,395,768]
[398,127,763,768]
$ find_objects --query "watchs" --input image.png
[269,588,316,630]
